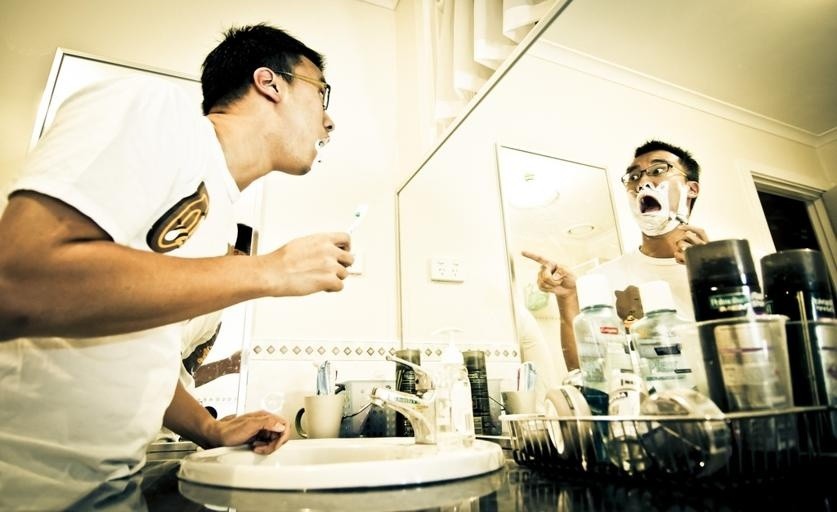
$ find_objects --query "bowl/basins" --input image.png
[496,412,731,483]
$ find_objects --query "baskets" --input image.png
[499,407,826,492]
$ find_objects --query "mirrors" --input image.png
[495,138,630,367]
[25,42,266,452]
[396,1,832,503]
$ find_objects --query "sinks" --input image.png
[177,437,509,511]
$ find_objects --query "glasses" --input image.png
[621,162,689,186]
[274,71,330,110]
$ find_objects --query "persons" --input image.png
[154,301,242,446]
[520,140,711,374]
[0,25,356,511]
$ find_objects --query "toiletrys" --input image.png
[571,276,643,398]
[626,283,701,395]
[432,328,476,439]
[600,337,649,451]
[683,238,799,468]
[646,391,730,470]
[538,386,598,456]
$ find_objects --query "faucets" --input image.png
[369,356,438,445]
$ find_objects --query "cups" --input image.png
[294,395,344,439]
[715,314,799,455]
[501,391,550,458]
[806,320,837,450]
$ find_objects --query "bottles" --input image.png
[432,338,476,451]
[573,304,638,399]
[682,238,769,418]
[633,307,700,401]
[756,247,837,405]
[393,349,425,439]
